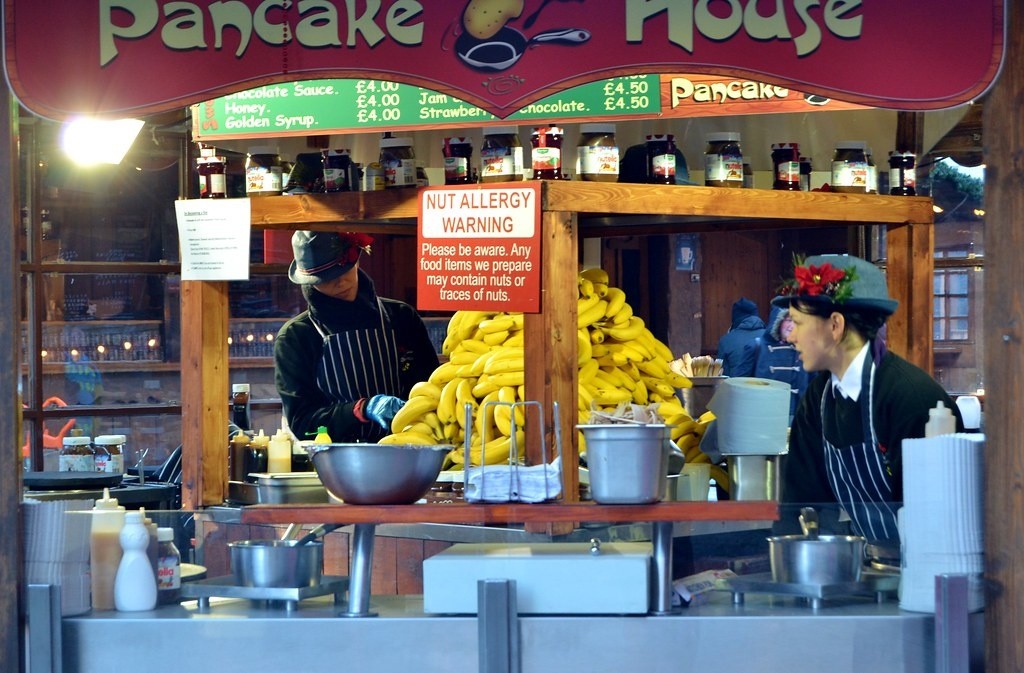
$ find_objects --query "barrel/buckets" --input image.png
[574,410,678,505]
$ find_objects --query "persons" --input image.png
[730,295,819,429]
[716,297,765,378]
[273,230,440,446]
[771,255,965,582]
[58,327,104,447]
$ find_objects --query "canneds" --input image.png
[59,433,125,477]
[155,527,180,605]
[197,122,922,199]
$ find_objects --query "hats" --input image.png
[770,252,899,314]
[288,229,374,285]
[731,297,758,329]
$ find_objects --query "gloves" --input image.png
[365,394,405,429]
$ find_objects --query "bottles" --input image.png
[87,488,181,612]
[195,126,920,201]
[924,401,957,437]
[58,435,129,474]
[232,383,252,433]
[230,428,293,483]
[314,426,332,472]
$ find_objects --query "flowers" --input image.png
[774,250,861,306]
[338,231,373,267]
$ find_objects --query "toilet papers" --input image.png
[705,376,792,454]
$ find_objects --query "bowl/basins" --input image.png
[304,444,453,504]
[226,539,324,608]
[765,534,868,607]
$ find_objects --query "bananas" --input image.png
[376,264,729,495]
